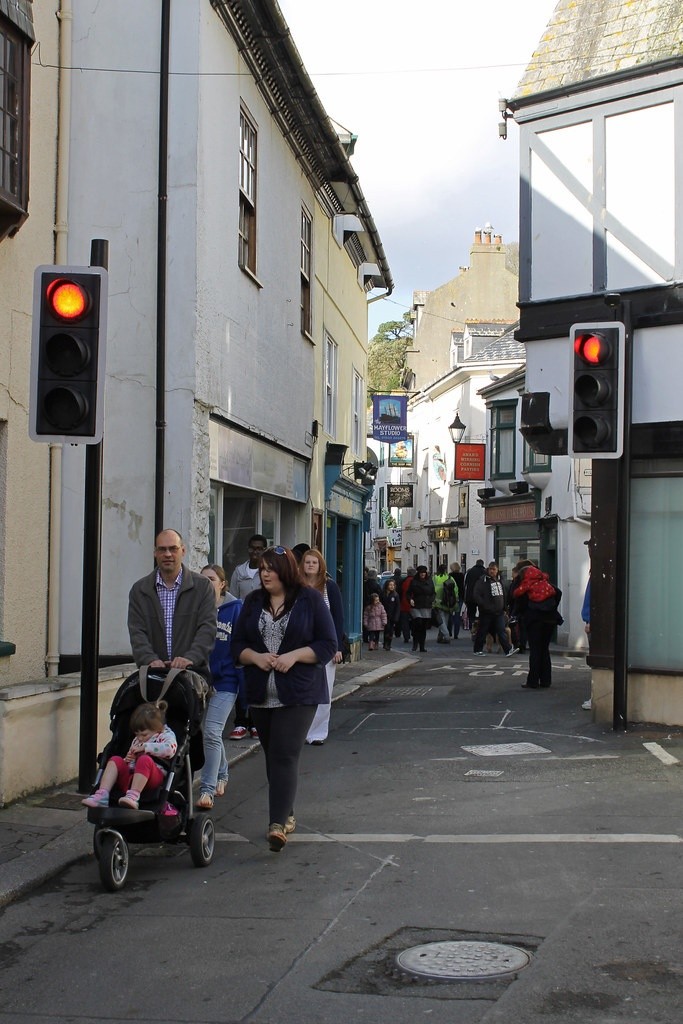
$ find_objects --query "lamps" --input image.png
[405,542,416,550]
[448,412,466,443]
[420,541,432,550]
[343,460,378,480]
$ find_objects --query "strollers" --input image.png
[87,664,217,894]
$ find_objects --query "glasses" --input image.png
[247,547,266,553]
[157,547,180,553]
[265,546,287,555]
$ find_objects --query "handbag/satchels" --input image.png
[431,596,443,628]
[338,633,352,664]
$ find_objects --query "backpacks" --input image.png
[440,575,456,608]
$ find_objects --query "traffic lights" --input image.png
[570,322,625,459]
[28,264,108,444]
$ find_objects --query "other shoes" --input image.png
[437,638,451,644]
[473,652,486,657]
[581,699,591,710]
[506,644,519,657]
[521,681,552,688]
[560,615,564,626]
[305,740,323,745]
[508,618,518,628]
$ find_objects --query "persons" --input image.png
[581,537,593,710]
[82,703,178,811]
[229,545,339,853]
[128,530,216,687]
[363,558,564,690]
[193,530,350,809]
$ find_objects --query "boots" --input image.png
[384,634,393,651]
[369,641,378,650]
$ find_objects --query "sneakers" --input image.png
[82,789,109,807]
[285,816,295,832]
[230,726,247,739]
[268,823,286,852]
[251,727,259,739]
[119,790,140,810]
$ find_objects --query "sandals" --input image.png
[195,793,214,809]
[215,779,227,797]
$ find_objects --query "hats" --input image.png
[416,565,427,573]
[584,538,591,545]
